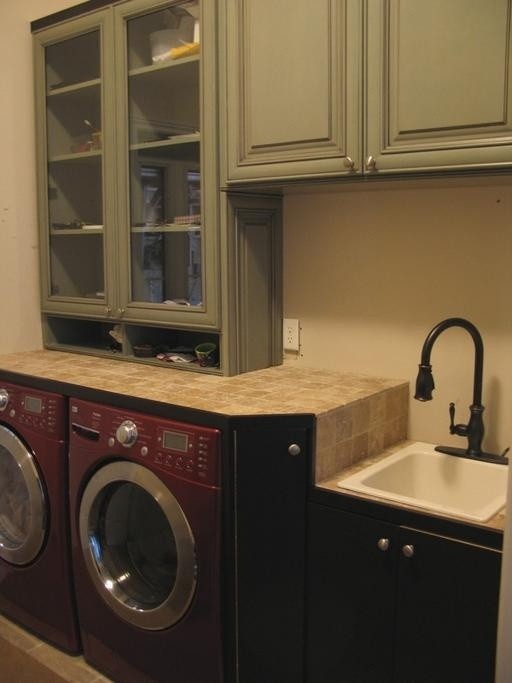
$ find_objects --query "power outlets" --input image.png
[282,318,300,353]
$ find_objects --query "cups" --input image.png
[84,133,102,151]
[194,343,218,366]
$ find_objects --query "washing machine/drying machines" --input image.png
[66,396,227,683]
[0,382,81,657]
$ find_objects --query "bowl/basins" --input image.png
[149,29,191,64]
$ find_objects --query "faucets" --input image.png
[413,317,509,465]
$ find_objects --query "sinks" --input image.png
[337,441,508,523]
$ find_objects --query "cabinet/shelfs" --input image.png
[303,486,505,681]
[30,1,283,376]
[216,0,511,184]
